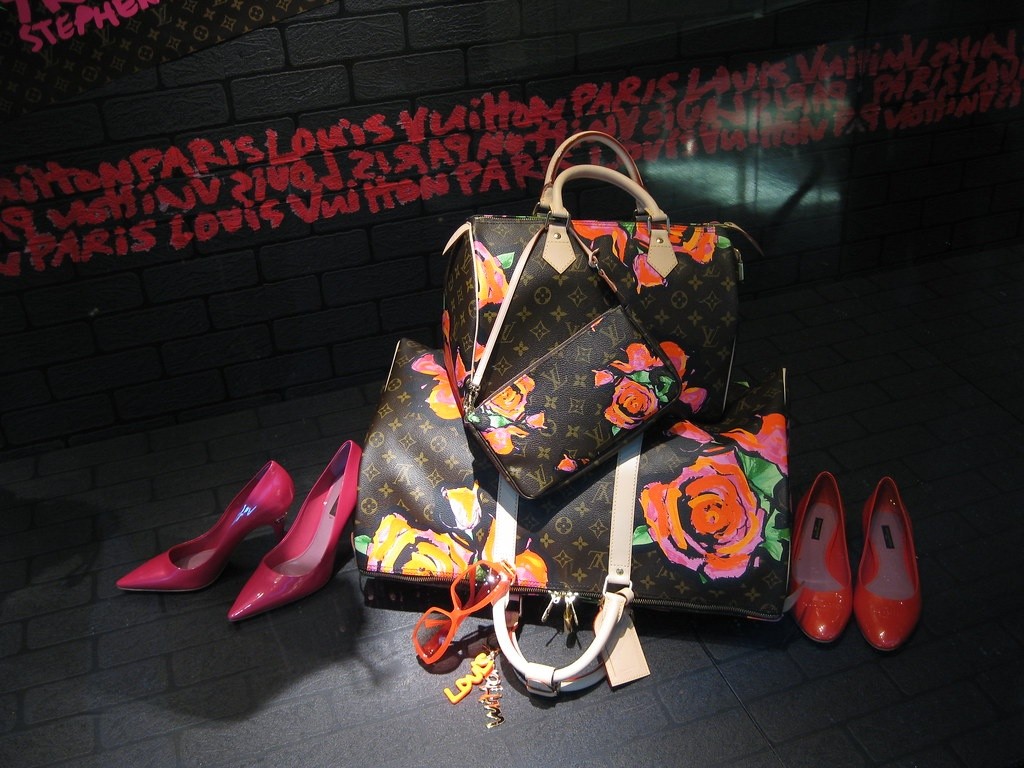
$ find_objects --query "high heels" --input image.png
[227,440,363,622]
[115,460,295,593]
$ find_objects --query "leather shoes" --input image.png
[854,477,922,652]
[791,471,854,643]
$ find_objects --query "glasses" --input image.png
[413,559,517,665]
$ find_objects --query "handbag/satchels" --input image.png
[350,335,796,697]
[461,223,683,499]
[442,130,766,423]
